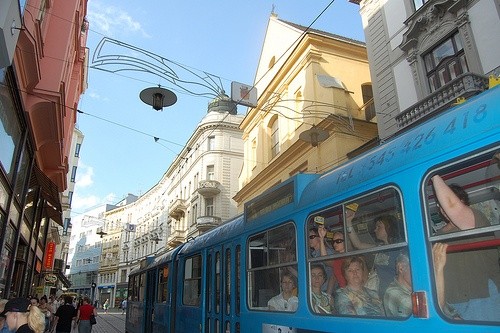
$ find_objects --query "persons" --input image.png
[334,255,385,316]
[104,298,109,316]
[26,294,52,333]
[382,242,449,317]
[0,296,45,332]
[120,297,127,314]
[55,293,100,309]
[310,263,335,314]
[428,174,500,322]
[50,295,77,333]
[317,224,369,288]
[307,216,338,296]
[0,298,16,333]
[73,296,97,332]
[49,295,58,332]
[345,205,406,299]
[266,271,298,311]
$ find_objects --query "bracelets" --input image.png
[346,223,354,232]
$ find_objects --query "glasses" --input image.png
[309,235,318,240]
[332,239,345,244]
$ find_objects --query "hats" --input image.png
[0,297,32,317]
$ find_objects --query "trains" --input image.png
[126,86,499,333]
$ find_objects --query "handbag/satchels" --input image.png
[90,305,97,325]
